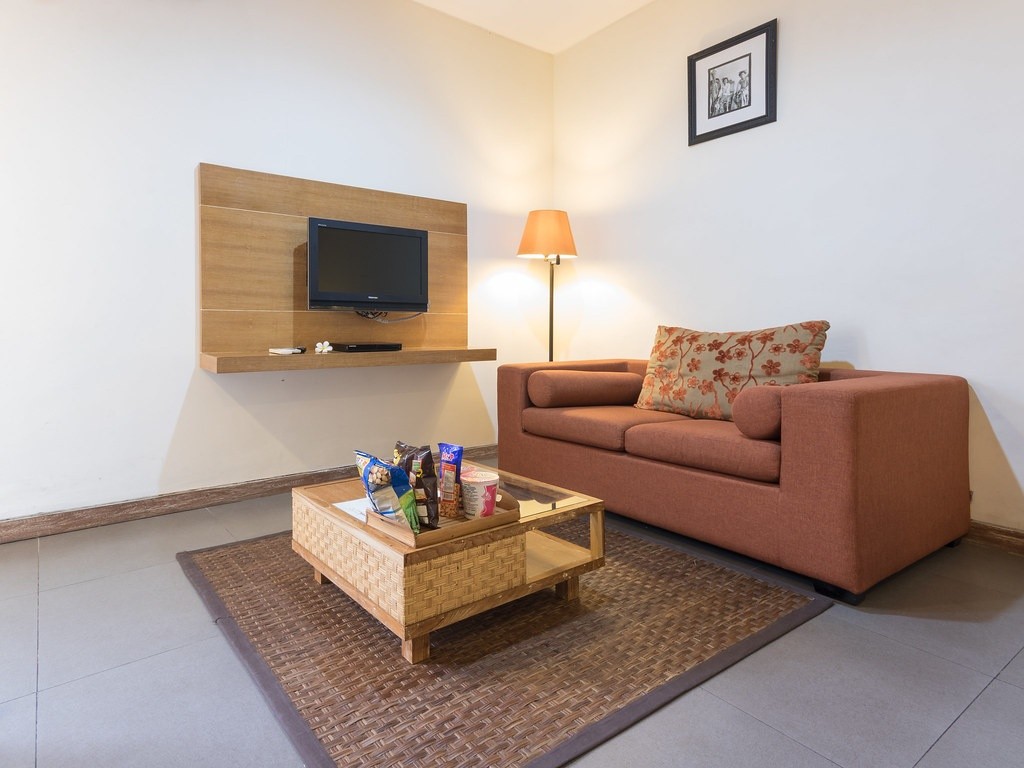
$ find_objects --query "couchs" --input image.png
[496,358,974,604]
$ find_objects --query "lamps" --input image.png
[517,210,578,361]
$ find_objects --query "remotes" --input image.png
[269,347,306,354]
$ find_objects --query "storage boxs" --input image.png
[363,488,521,550]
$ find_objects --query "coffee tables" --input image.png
[291,456,609,666]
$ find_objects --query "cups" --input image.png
[460,472,499,519]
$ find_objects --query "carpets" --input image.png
[177,516,838,768]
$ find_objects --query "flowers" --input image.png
[315,340,333,354]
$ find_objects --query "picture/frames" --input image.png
[687,17,777,147]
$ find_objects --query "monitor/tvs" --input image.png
[307,218,428,312]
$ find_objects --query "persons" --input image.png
[710,70,750,116]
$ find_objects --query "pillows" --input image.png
[525,368,643,409]
[731,384,785,439]
[635,319,832,421]
